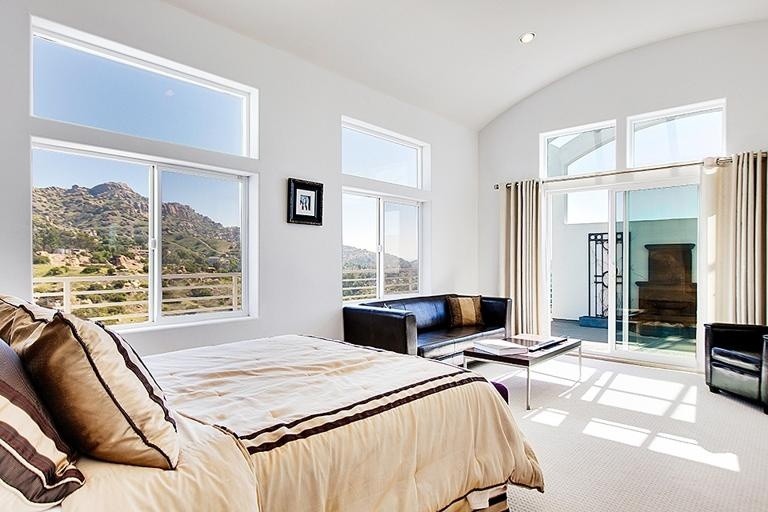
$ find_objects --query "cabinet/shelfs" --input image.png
[645,243,694,284]
[635,281,697,338]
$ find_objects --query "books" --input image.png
[473,339,528,356]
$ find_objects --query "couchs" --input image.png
[704,322,768,415]
[342,294,513,370]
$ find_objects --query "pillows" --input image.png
[0,339,86,512]
[0,294,181,468]
[446,295,485,329]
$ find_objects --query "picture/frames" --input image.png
[287,178,325,227]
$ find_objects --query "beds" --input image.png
[1,332,545,512]
